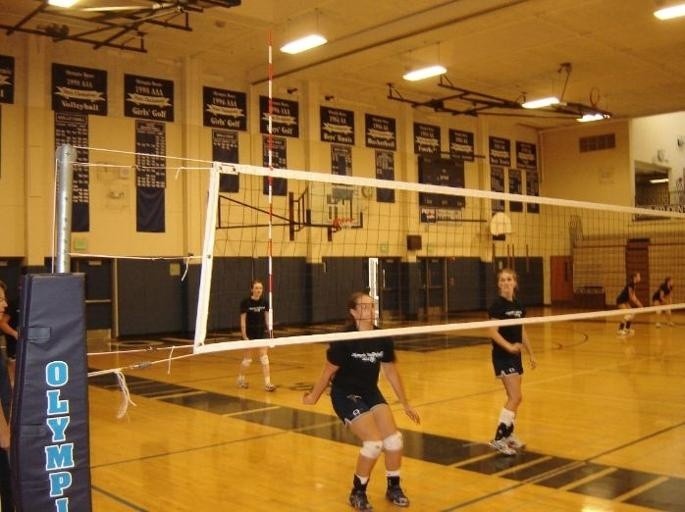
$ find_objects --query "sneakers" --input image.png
[234,376,277,393]
[488,435,527,457]
[655,320,675,328]
[615,328,634,335]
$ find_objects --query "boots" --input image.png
[350,474,376,512]
[385,476,408,507]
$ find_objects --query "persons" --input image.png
[652,276,678,326]
[486,268,538,456]
[234,278,278,391]
[300,292,421,511]
[0,294,20,387]
[0,280,18,512]
[615,271,644,335]
